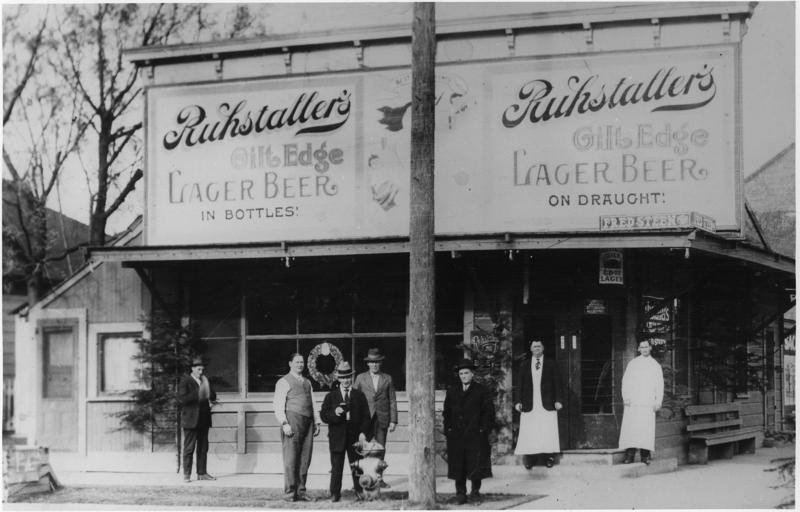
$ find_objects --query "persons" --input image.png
[617,335,665,466]
[180,356,218,482]
[440,357,499,504]
[509,336,564,469]
[319,360,373,503]
[273,353,322,501]
[350,348,398,488]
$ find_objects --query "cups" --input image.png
[339,403,348,416]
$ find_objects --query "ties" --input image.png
[343,387,349,413]
[199,377,206,403]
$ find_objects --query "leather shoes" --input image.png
[545,454,555,467]
[197,472,217,481]
[284,496,295,503]
[523,456,535,469]
[183,473,192,482]
[296,492,312,501]
[332,492,341,503]
[354,488,364,501]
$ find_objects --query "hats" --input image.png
[363,348,386,363]
[333,361,357,379]
[453,358,479,373]
[188,355,208,368]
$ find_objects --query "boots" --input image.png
[640,449,651,465]
[623,449,636,464]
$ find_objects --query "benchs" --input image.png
[682,401,759,462]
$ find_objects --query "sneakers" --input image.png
[468,488,481,501]
[455,490,468,504]
[380,480,391,489]
